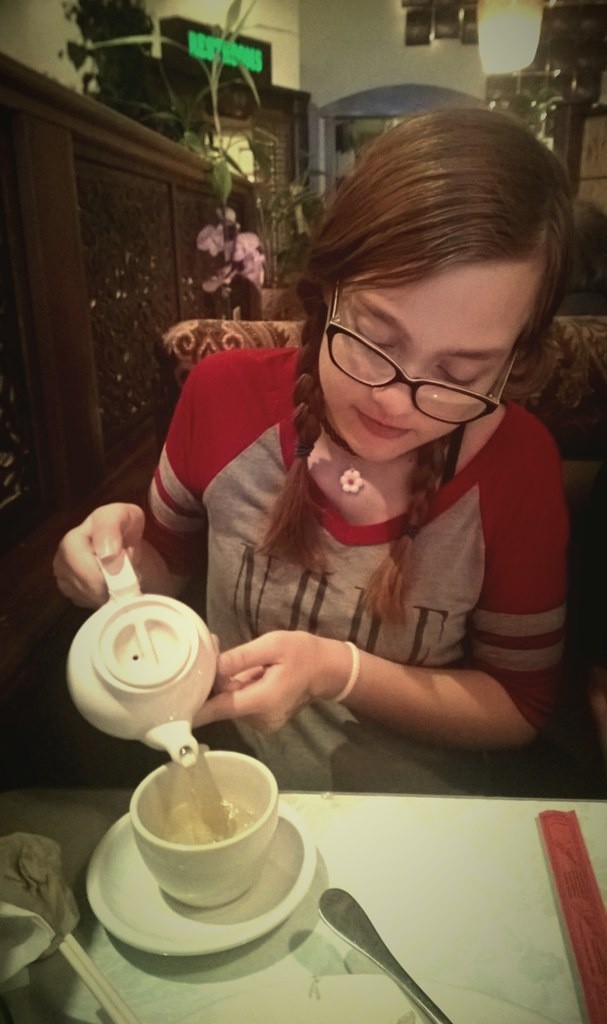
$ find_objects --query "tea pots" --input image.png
[65,548,217,766]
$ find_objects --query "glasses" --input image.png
[322,283,521,425]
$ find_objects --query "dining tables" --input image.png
[2,785,606,1024]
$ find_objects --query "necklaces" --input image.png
[320,416,365,495]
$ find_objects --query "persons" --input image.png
[568,463,607,758]
[55,105,573,797]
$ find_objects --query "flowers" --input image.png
[198,205,269,297]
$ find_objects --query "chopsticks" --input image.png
[59,931,140,1024]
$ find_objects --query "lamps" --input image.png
[405,5,485,47]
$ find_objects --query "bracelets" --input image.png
[319,641,362,707]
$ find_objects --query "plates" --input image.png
[85,802,316,956]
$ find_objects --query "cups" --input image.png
[130,751,279,906]
